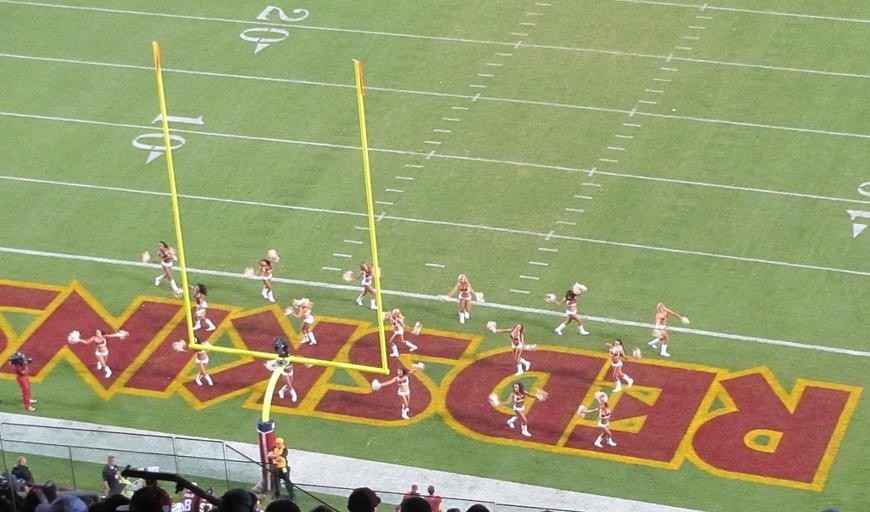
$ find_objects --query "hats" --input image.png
[348,488,380,511]
[35,495,88,512]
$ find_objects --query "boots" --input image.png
[402,407,410,419]
[648,338,671,357]
[516,358,530,376]
[194,318,216,331]
[301,331,317,346]
[155,275,165,286]
[506,416,532,436]
[389,345,399,357]
[197,372,213,386]
[554,323,566,335]
[278,384,298,402]
[594,435,617,448]
[261,287,275,302]
[170,279,182,293]
[356,293,378,310]
[459,311,469,324]
[97,361,112,378]
[405,341,418,351]
[613,374,634,392]
[578,325,589,335]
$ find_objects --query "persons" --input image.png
[496,323,531,375]
[577,391,617,448]
[289,298,319,345]
[386,307,419,357]
[379,368,418,419]
[0,454,495,511]
[76,327,125,378]
[188,283,216,331]
[353,262,380,310]
[606,338,639,392]
[498,382,541,437]
[268,438,292,496]
[449,275,478,324]
[545,283,589,336]
[151,240,186,295]
[648,302,683,356]
[251,258,274,302]
[9,352,39,411]
[277,354,310,402]
[182,336,214,386]
